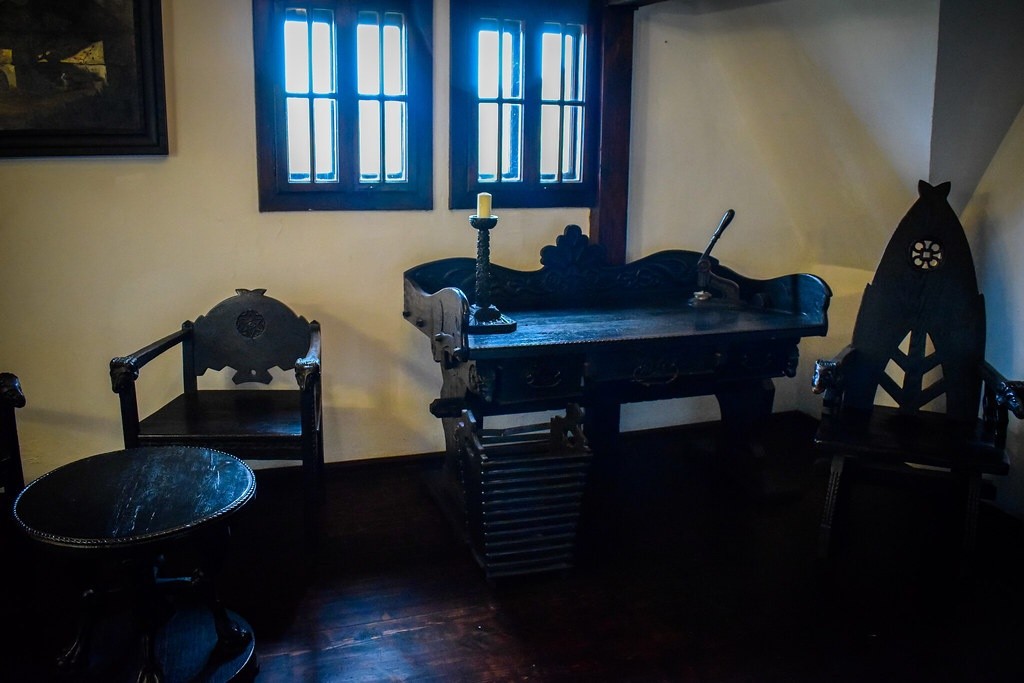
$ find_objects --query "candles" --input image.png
[477,192,492,218]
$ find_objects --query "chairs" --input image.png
[811,178,1024,587]
[110,289,324,570]
[0,373,34,617]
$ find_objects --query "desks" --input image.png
[404,225,833,580]
[14,446,261,683]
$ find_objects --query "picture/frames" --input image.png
[0,0,170,157]
